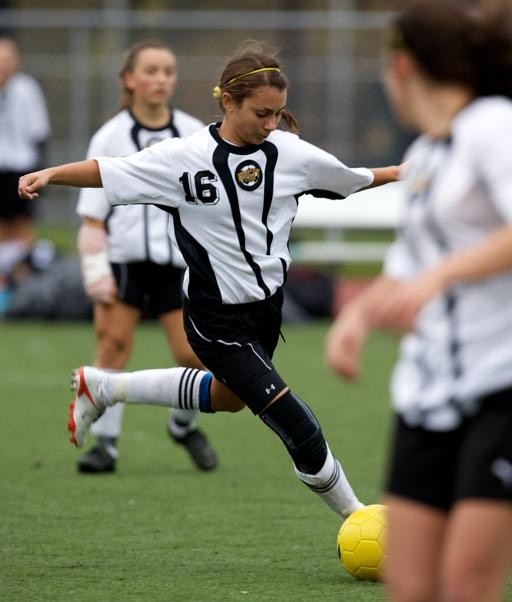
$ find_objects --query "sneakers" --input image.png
[66,364,110,452]
[73,428,122,473]
[164,418,220,471]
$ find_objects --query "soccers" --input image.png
[336,504,389,582]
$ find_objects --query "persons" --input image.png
[19,38,408,519]
[76,38,218,474]
[0,26,52,294]
[322,0,512,602]
